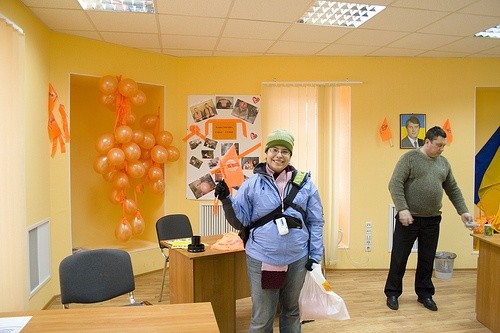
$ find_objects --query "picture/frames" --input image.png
[399,114,426,149]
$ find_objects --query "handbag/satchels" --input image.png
[298,263,350,322]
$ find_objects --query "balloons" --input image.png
[93,75,180,242]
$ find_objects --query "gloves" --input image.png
[305,259,318,271]
[214,179,230,201]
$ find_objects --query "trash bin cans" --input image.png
[435,251,456,279]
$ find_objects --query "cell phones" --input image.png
[275,217,289,235]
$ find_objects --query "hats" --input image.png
[265,129,295,156]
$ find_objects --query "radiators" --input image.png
[199,204,240,237]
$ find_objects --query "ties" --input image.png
[414,141,418,148]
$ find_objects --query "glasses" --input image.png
[268,148,291,156]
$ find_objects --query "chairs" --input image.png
[155,214,194,302]
[59,248,135,309]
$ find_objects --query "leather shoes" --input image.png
[417,295,437,311]
[386,296,398,310]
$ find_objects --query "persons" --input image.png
[192,98,258,195]
[402,116,425,147]
[384,126,474,312]
[214,130,324,333]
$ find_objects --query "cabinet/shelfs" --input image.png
[0,302,221,333]
[470,232,500,333]
[160,234,251,333]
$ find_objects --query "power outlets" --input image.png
[365,223,372,252]
